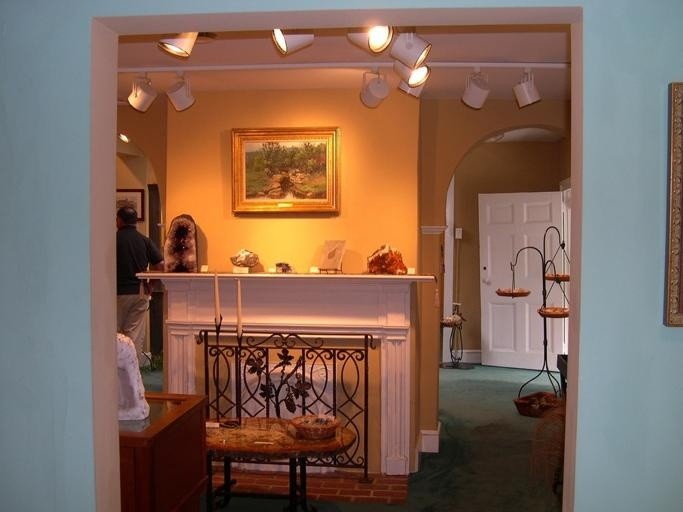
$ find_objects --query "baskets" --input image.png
[290,415,341,439]
[514,392,554,418]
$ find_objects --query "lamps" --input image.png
[402,80,426,98]
[360,65,388,108]
[461,65,492,109]
[165,72,196,112]
[388,26,430,69]
[512,66,541,110]
[155,31,201,58]
[126,70,157,110]
[272,30,313,56]
[394,58,429,86]
[347,28,392,56]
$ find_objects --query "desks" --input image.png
[205,419,357,512]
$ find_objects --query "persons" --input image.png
[116,207,164,368]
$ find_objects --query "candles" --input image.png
[214,273,221,326]
[235,277,243,336]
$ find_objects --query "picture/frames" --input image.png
[116,189,144,223]
[231,127,341,216]
[665,82,683,328]
